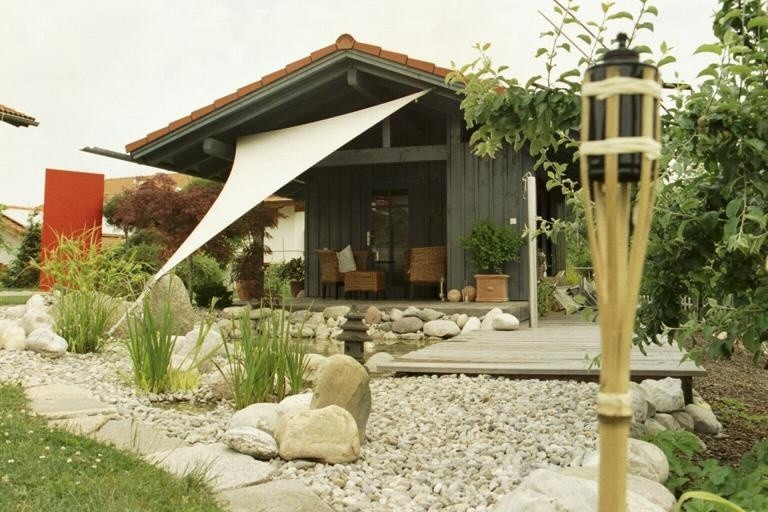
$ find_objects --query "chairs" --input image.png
[402,246,446,301]
[313,248,370,299]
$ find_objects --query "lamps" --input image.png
[585,31,660,178]
[337,305,372,366]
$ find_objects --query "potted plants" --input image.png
[273,256,306,297]
[229,239,272,301]
[454,217,527,302]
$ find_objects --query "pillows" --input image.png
[323,244,357,273]
